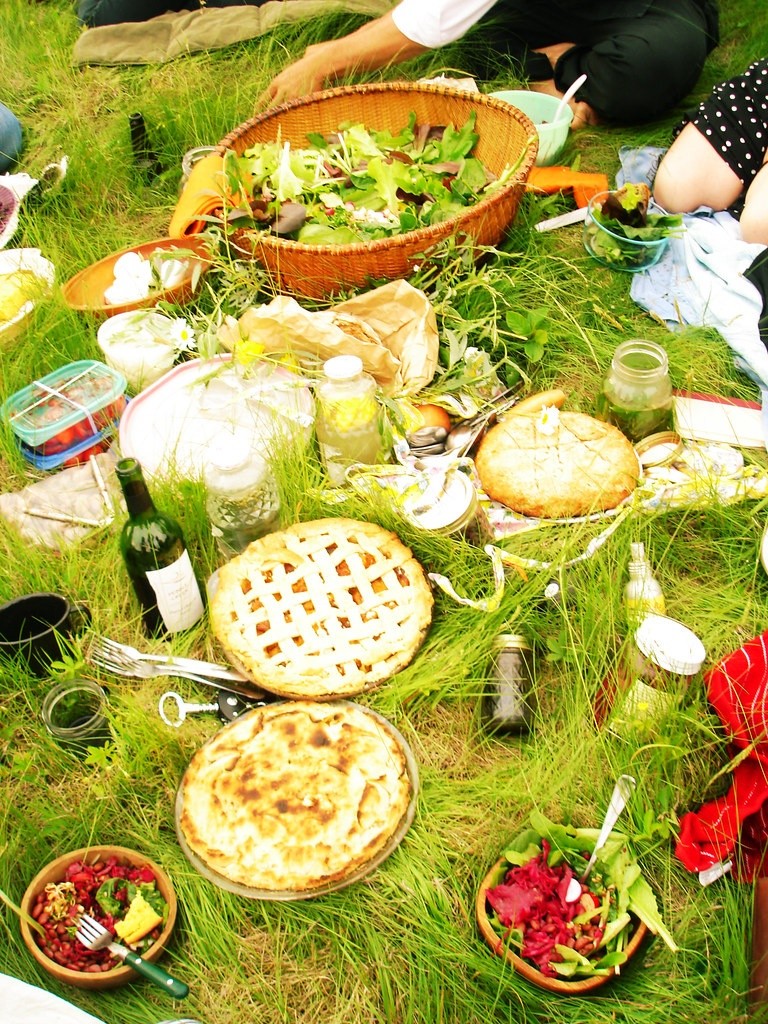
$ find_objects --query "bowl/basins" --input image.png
[61,234,219,322]
[97,311,179,389]
[0,360,128,455]
[15,394,132,477]
[477,856,645,995]
[21,845,177,987]
[0,248,55,346]
[487,90,573,167]
[583,192,672,272]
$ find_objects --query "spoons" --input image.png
[411,380,521,454]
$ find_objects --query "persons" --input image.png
[252,0,721,135]
[649,52,768,248]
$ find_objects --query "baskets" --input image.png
[183,78,539,304]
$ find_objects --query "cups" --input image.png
[0,594,92,679]
[42,679,119,768]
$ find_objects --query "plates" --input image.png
[175,698,419,901]
[205,555,434,701]
[495,444,643,523]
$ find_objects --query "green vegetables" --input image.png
[589,184,687,267]
[485,809,680,975]
[231,111,536,245]
[55,877,169,971]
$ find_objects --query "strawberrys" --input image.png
[12,374,127,470]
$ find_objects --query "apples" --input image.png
[409,403,450,432]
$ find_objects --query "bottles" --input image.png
[315,354,381,490]
[203,434,279,563]
[115,458,205,643]
[623,542,665,629]
[128,113,164,187]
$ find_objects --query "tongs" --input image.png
[21,456,114,528]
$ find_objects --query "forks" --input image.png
[89,633,267,699]
[75,913,189,1000]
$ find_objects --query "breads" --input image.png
[505,389,565,414]
[0,270,41,325]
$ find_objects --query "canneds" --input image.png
[591,617,705,743]
[481,634,533,734]
[597,338,673,444]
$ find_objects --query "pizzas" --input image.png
[476,410,639,518]
[180,701,411,892]
[206,516,433,697]
